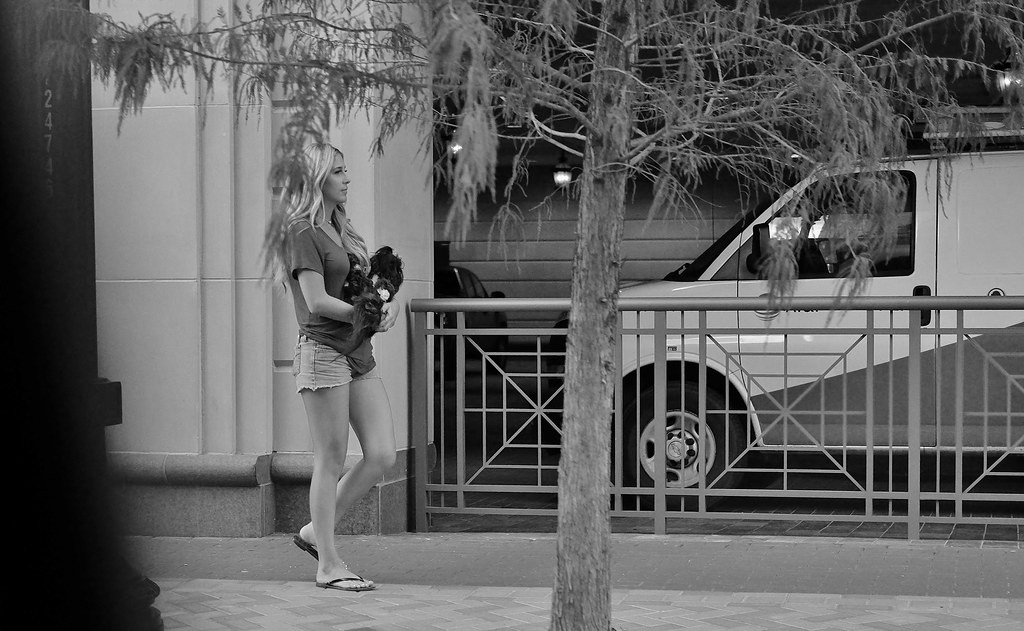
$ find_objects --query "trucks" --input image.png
[545,148,1023,519]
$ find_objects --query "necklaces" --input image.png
[318,213,331,223]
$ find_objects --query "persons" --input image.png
[264,145,401,590]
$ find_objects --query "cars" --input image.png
[434,266,510,379]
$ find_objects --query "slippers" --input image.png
[293,535,319,561]
[316,575,375,591]
